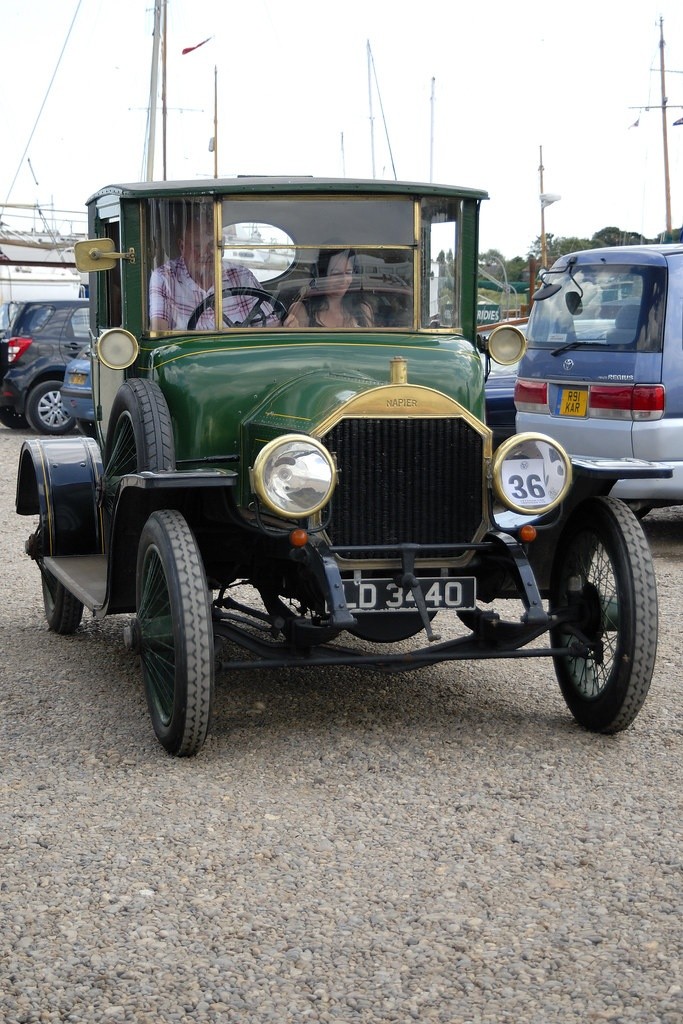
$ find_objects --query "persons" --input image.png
[283,238,374,328]
[149,213,281,331]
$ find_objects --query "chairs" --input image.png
[28,307,74,338]
[606,304,641,345]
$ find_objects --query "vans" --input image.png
[513,243,683,520]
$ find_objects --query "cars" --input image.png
[0,298,98,438]
[481,318,617,441]
[13,173,660,757]
